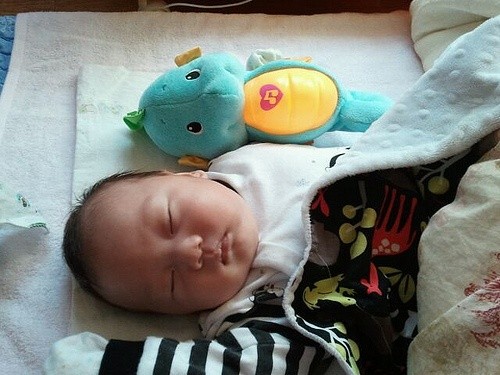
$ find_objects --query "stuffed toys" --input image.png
[124,44,396,169]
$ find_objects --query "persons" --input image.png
[42,141,355,375]
